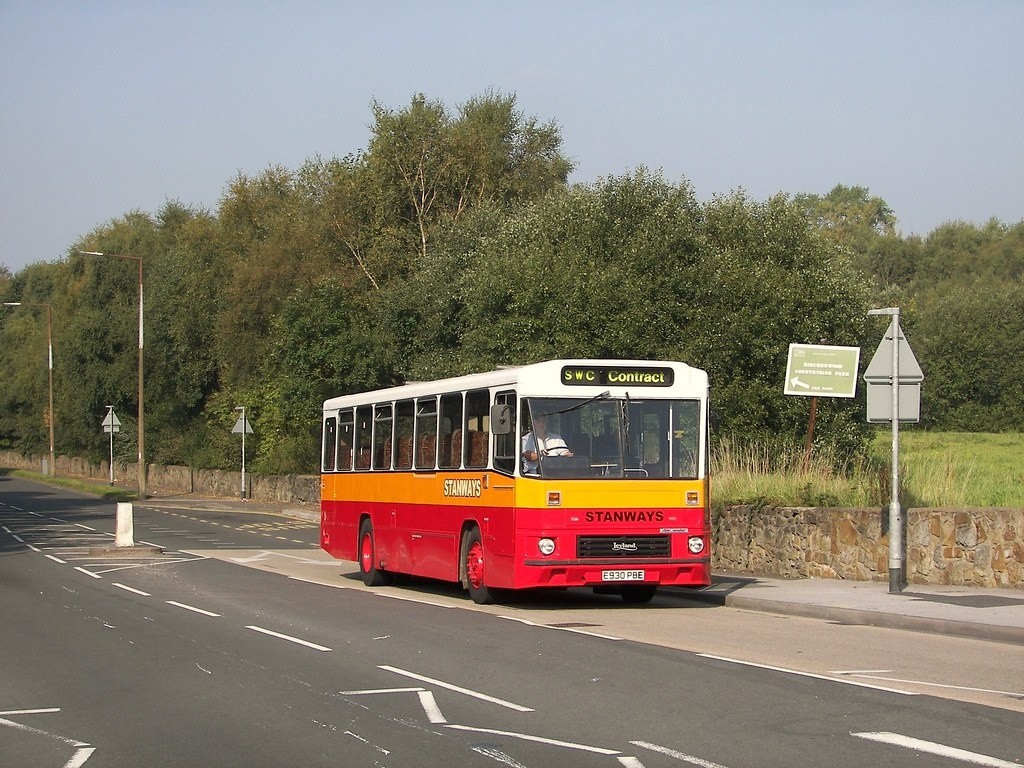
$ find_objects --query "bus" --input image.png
[320,358,711,605]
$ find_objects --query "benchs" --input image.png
[329,430,635,472]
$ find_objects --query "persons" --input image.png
[521,411,575,473]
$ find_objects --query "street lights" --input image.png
[79,250,147,500]
[3,302,57,479]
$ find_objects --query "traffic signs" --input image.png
[784,343,860,399]
